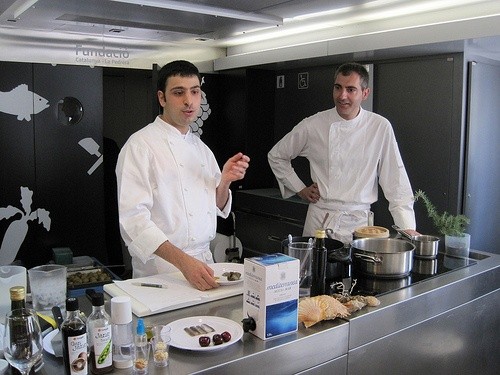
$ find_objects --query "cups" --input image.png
[129,343,152,375]
[151,325,171,335]
[150,335,171,368]
[288,241,312,296]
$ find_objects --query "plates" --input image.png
[206,263,245,285]
[43,328,63,358]
[162,315,244,352]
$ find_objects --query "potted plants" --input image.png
[413,191,470,257]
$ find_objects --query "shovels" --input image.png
[51,306,65,357]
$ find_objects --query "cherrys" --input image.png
[213,332,231,345]
[199,336,210,347]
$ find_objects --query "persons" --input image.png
[267,63,417,244]
[115,59,251,291]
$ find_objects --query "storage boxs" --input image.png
[242,253,299,341]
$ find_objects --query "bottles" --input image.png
[111,296,134,368]
[310,230,325,298]
[84,288,114,375]
[61,297,91,375]
[133,319,147,342]
[9,284,37,375]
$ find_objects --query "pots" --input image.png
[280,236,351,297]
[349,237,416,279]
[393,225,440,259]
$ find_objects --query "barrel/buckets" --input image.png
[352,227,390,241]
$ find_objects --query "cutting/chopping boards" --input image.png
[102,271,244,318]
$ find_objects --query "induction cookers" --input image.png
[352,251,478,297]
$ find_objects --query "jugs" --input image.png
[27,265,67,319]
[0,265,26,324]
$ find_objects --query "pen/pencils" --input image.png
[132,283,168,289]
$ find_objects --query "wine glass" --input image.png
[3,308,43,375]
